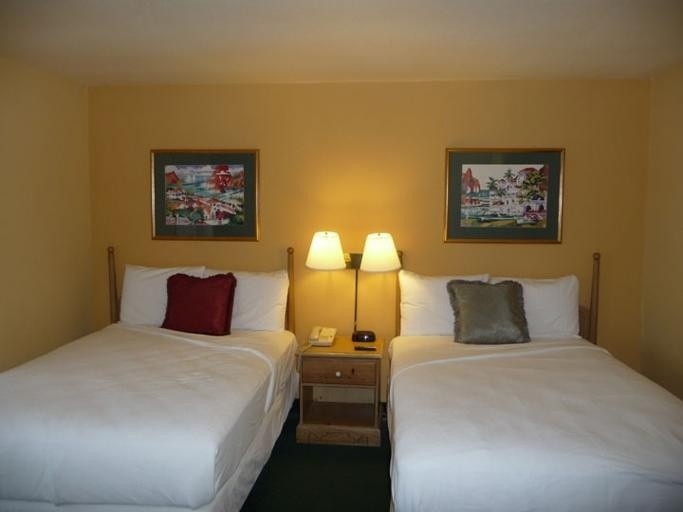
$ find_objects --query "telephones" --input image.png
[308,326,337,347]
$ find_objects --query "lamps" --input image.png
[358,231,401,272]
[304,230,348,273]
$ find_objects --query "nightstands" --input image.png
[296,333,382,450]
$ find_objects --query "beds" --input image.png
[1,247,299,511]
[388,253,683,512]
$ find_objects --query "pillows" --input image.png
[397,272,489,337]
[206,268,289,333]
[446,279,528,341]
[493,276,578,337]
[120,266,205,328]
[159,273,235,334]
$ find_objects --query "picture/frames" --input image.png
[150,148,259,242]
[445,146,563,242]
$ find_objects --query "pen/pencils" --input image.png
[353,346,376,351]
